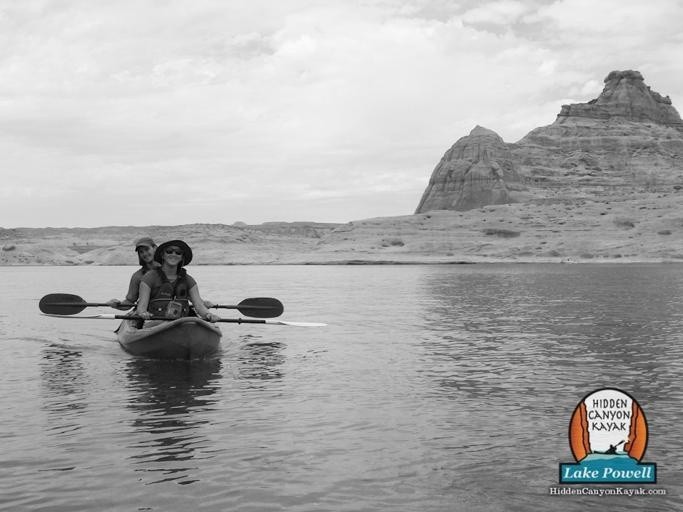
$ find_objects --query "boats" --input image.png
[119,304,224,356]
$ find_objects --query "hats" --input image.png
[153,239,193,266]
[135,237,154,252]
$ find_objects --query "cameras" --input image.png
[166,302,183,318]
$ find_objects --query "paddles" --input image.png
[39,294,283,318]
[39,311,326,328]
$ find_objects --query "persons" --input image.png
[107,237,213,311]
[136,240,220,329]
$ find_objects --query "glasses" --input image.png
[165,246,184,255]
